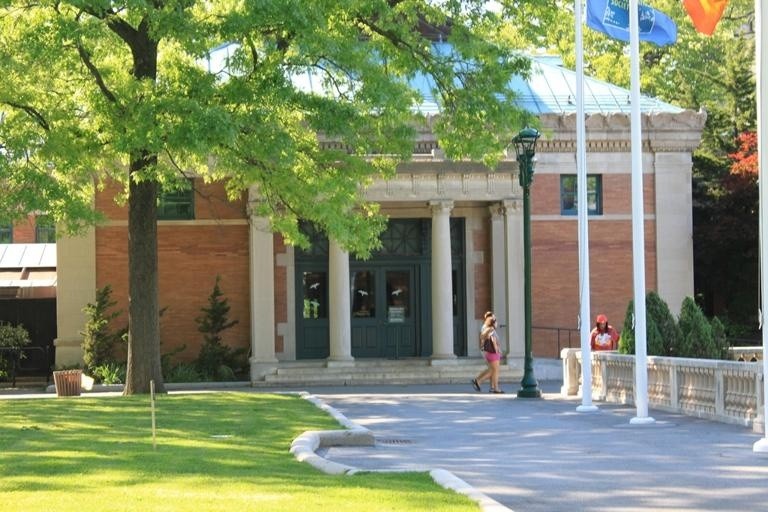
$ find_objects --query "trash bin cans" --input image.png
[53,369,82,396]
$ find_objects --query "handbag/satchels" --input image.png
[484,336,495,353]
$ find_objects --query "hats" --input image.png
[597,314,608,323]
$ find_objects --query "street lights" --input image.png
[511,131,541,398]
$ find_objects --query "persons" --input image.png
[471,311,500,392]
[590,313,619,351]
[472,318,505,393]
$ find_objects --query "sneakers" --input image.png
[471,378,481,392]
[489,390,505,394]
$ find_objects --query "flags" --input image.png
[684,0,731,36]
[587,1,678,48]
